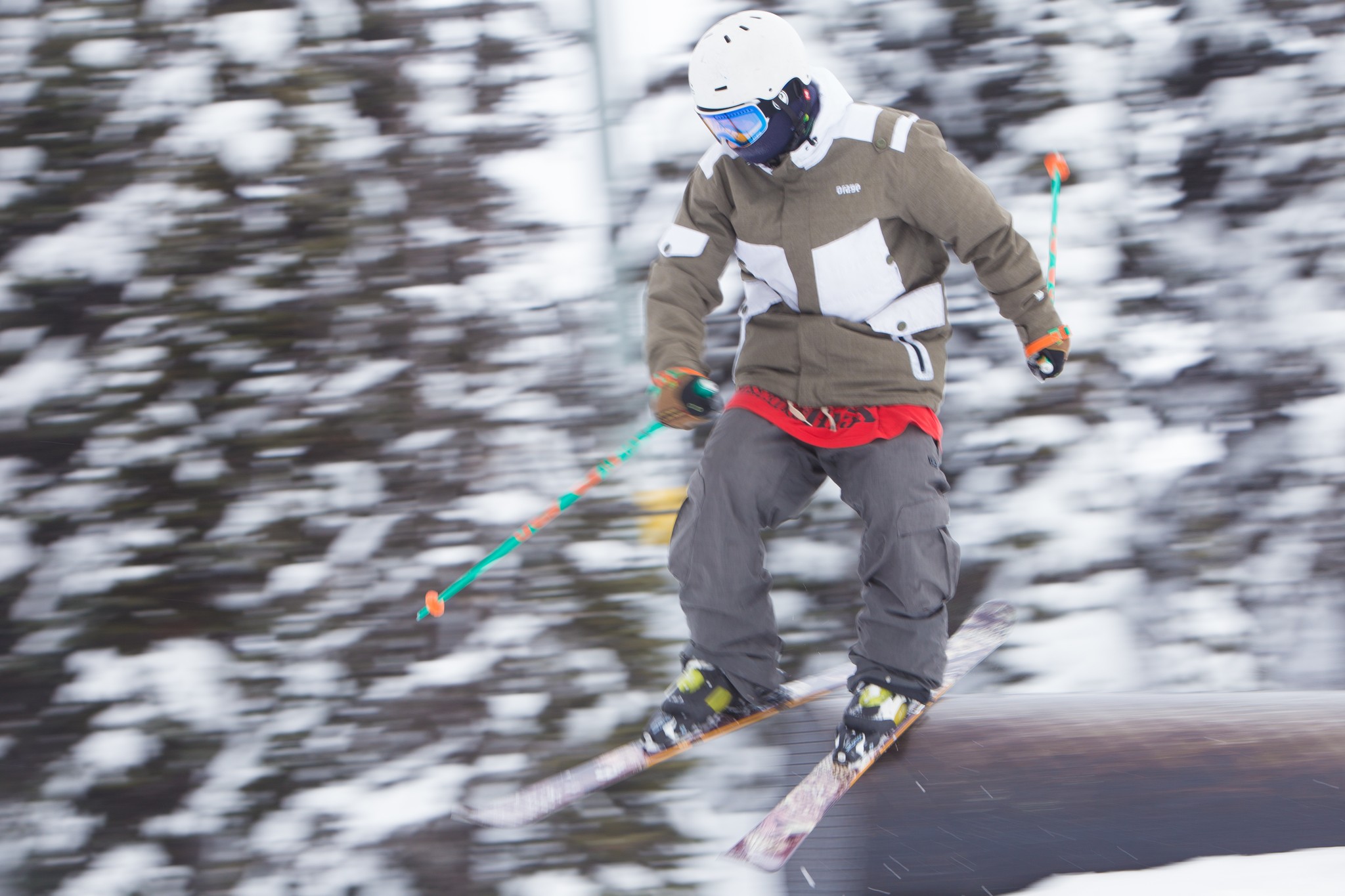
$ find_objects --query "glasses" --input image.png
[693,99,775,149]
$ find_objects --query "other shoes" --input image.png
[834,682,912,763]
[643,657,788,753]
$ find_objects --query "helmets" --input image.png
[688,11,839,159]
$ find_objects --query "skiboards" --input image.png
[451,598,1017,875]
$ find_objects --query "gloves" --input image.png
[647,368,725,429]
[1024,325,1070,382]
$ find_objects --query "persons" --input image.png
[639,9,1071,773]
